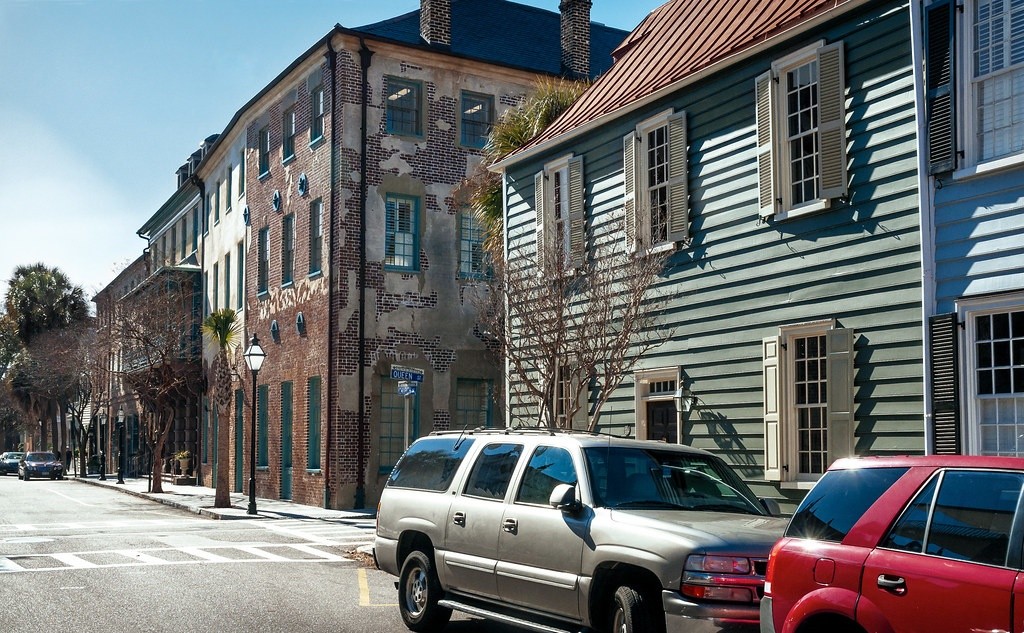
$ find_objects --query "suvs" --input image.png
[371,423,787,633]
[758,457,1024,633]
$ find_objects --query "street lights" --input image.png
[98,405,126,484]
[243,332,267,514]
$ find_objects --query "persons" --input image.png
[9,440,73,474]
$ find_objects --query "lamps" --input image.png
[672,375,696,413]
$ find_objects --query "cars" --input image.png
[0,451,64,481]
